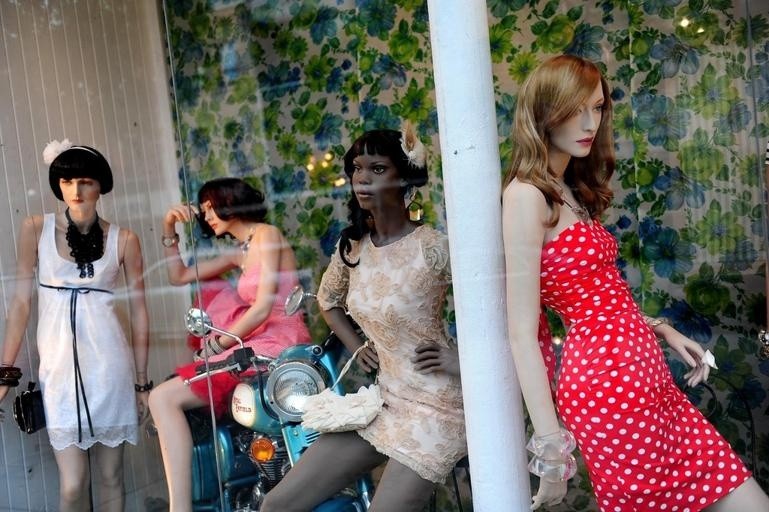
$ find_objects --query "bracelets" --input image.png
[1,367,22,388]
[525,429,579,481]
[135,379,154,393]
[648,316,674,344]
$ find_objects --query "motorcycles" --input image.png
[184,285,377,512]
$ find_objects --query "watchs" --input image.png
[161,234,181,247]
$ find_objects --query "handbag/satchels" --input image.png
[299,384,384,433]
[11,382,46,433]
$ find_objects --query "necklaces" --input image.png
[65,205,105,278]
[237,220,258,273]
[562,197,592,225]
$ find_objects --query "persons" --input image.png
[501,54,767,511]
[260,115,467,512]
[146,177,313,512]
[1,137,149,511]
